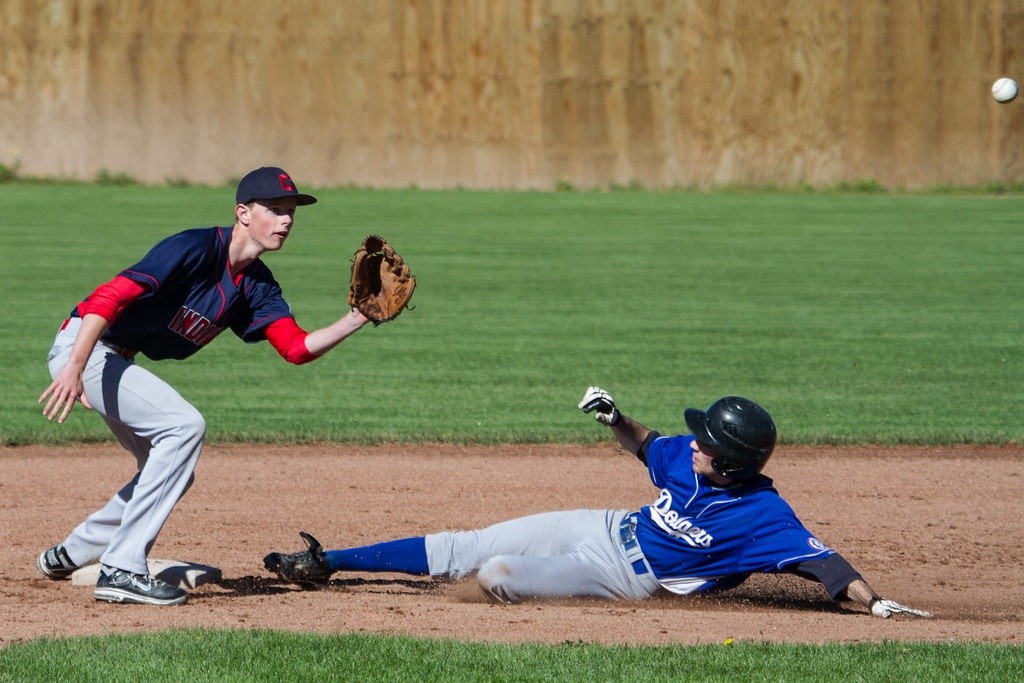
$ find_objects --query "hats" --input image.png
[236,167,318,206]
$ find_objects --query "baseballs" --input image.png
[991,76,1022,105]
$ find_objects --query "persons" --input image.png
[261,386,932,619]
[39,167,415,606]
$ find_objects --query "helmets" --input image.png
[683,396,777,484]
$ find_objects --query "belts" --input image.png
[59,318,136,360]
[619,511,648,575]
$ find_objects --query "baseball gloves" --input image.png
[344,233,420,329]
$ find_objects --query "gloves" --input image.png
[867,597,933,619]
[577,386,621,427]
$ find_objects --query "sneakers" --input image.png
[37,544,82,581]
[263,532,337,591]
[94,563,189,606]
